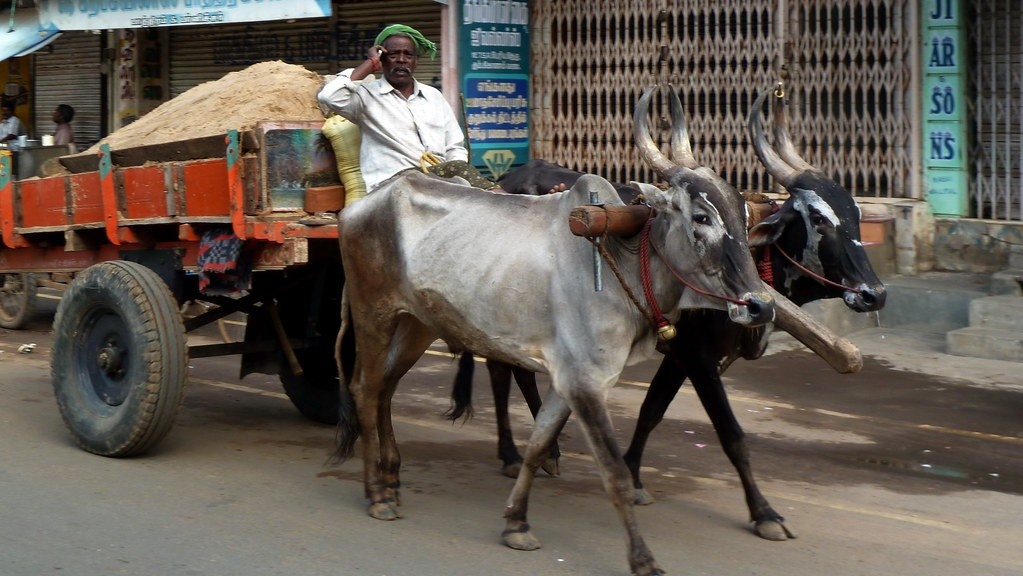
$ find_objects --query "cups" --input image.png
[19,135,28,147]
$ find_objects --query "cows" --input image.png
[339,83,888,576]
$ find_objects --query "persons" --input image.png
[0,107,29,144]
[316,25,469,194]
[51,103,76,145]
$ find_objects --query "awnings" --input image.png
[0,6,66,61]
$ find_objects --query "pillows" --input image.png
[321,116,367,207]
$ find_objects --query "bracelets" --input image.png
[368,56,381,72]
